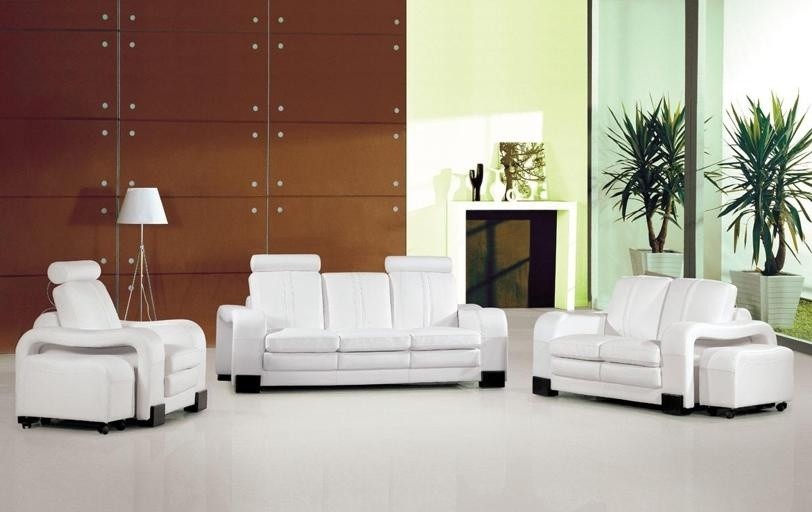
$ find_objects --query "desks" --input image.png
[449,199,574,308]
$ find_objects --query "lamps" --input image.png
[113,184,173,321]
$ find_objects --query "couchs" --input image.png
[212,252,508,393]
[529,271,777,418]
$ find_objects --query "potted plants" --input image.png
[717,91,812,330]
[605,89,729,278]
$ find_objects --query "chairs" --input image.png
[12,254,209,433]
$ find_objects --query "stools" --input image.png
[13,349,135,437]
[698,343,794,416]
